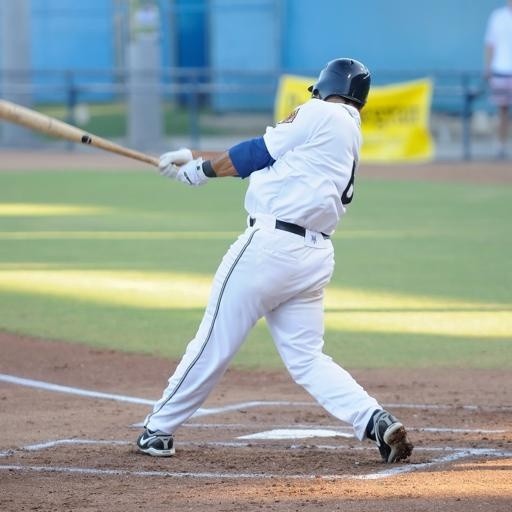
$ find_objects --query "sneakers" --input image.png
[136,429,176,457]
[372,409,413,464]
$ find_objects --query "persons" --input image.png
[134,57,414,464]
[484,1,512,159]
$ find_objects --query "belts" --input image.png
[249,217,329,242]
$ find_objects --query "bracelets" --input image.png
[202,160,218,178]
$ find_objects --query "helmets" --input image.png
[308,57,371,110]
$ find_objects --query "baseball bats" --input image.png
[1,100,182,175]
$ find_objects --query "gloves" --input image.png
[175,156,209,188]
[158,148,194,178]
